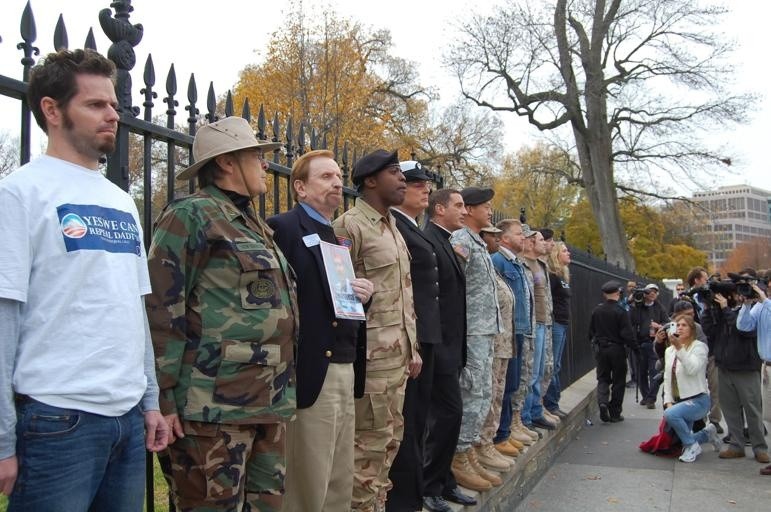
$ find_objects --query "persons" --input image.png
[480,219,574,457]
[586,266,770,477]
[264,150,375,512]
[385,161,440,511]
[148,116,300,512]
[449,187,503,491]
[331,150,417,512]
[422,187,477,510]
[0,47,170,512]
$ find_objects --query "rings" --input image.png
[361,296,366,303]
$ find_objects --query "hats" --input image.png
[349,148,399,186]
[539,229,554,239]
[520,224,537,238]
[645,284,659,291]
[175,116,286,181]
[480,223,503,234]
[458,186,495,206]
[601,281,622,293]
[399,160,431,182]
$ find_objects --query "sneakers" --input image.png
[754,450,769,463]
[420,399,569,512]
[599,403,610,422]
[759,465,771,475]
[719,448,745,459]
[610,415,624,423]
[646,398,656,409]
[704,421,724,453]
[678,439,703,463]
[640,399,647,405]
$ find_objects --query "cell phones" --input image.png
[670,322,676,336]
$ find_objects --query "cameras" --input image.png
[739,282,759,299]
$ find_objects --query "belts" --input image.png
[760,359,771,366]
